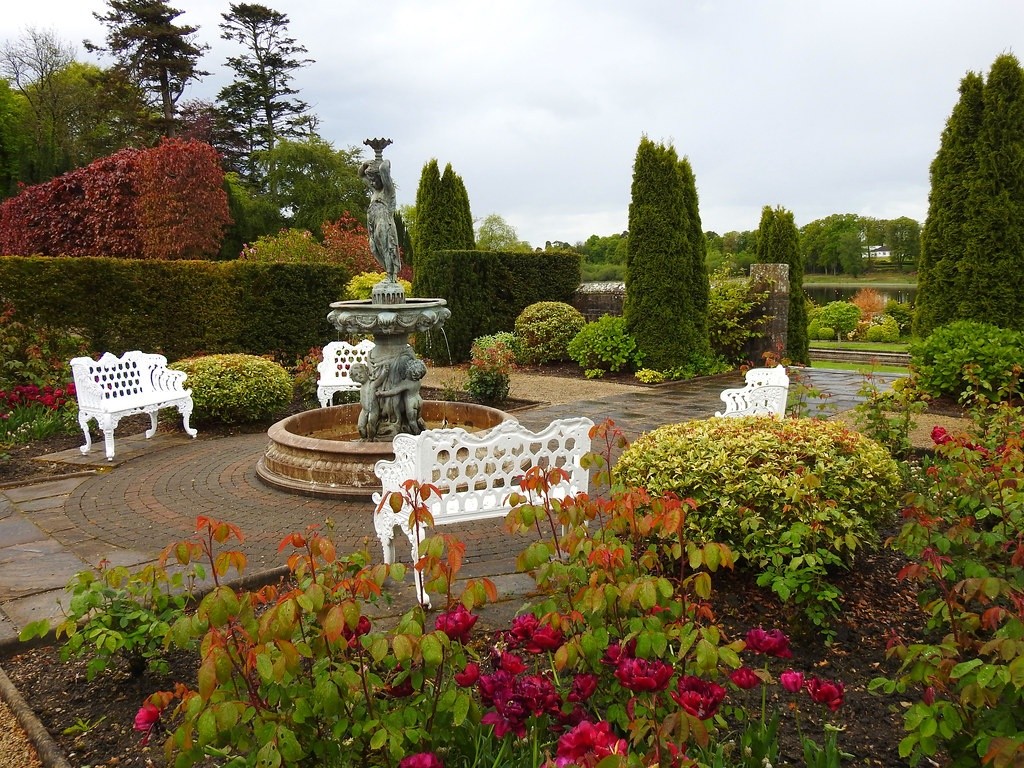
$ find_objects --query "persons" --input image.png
[358,160,401,284]
[350,363,390,442]
[375,359,427,434]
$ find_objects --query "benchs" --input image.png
[371,417,596,606]
[316,339,376,407]
[70,349,198,463]
[714,363,789,421]
